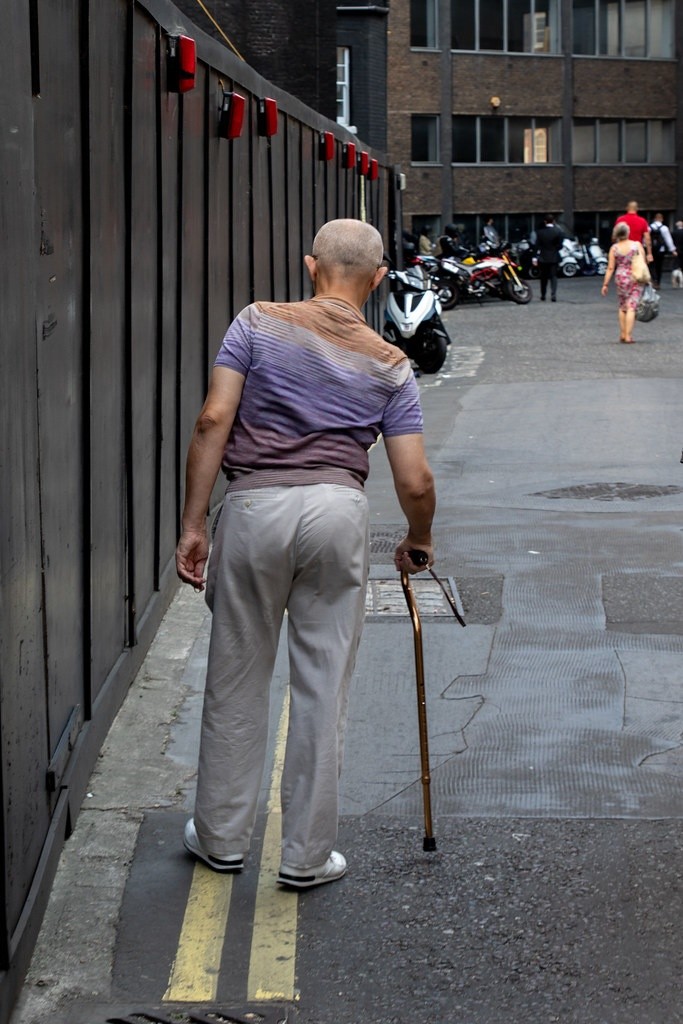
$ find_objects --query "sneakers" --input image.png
[182,817,245,871]
[277,852,348,887]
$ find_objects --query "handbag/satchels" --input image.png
[630,241,650,284]
[635,283,660,323]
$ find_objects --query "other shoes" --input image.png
[624,338,636,344]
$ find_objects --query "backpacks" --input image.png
[648,224,667,256]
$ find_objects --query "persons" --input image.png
[419,226,432,255]
[532,214,564,302]
[602,202,677,344]
[174,219,437,889]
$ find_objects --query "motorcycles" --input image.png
[382,251,452,375]
[402,226,609,312]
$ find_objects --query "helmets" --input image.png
[444,224,459,238]
[420,224,433,236]
